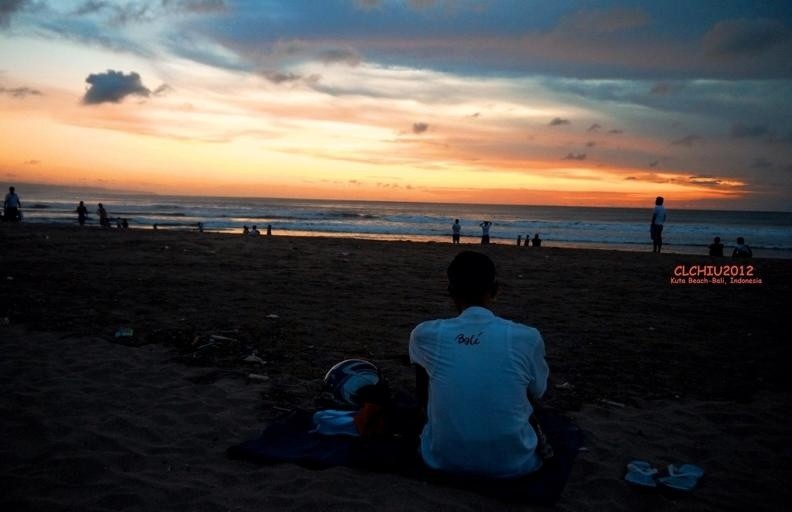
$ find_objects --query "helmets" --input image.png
[317,357,380,409]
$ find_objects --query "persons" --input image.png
[104,218,111,227]
[732,236,753,260]
[267,224,271,235]
[480,221,492,244]
[532,233,541,246]
[649,197,667,253]
[77,201,89,226]
[96,204,107,226]
[116,217,121,228]
[122,220,129,228]
[251,225,260,235]
[525,235,529,246]
[243,225,248,234]
[406,250,560,495]
[518,235,522,246]
[709,236,724,256]
[452,219,461,244]
[3,186,21,224]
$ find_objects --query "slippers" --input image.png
[661,464,706,492]
[624,458,659,490]
